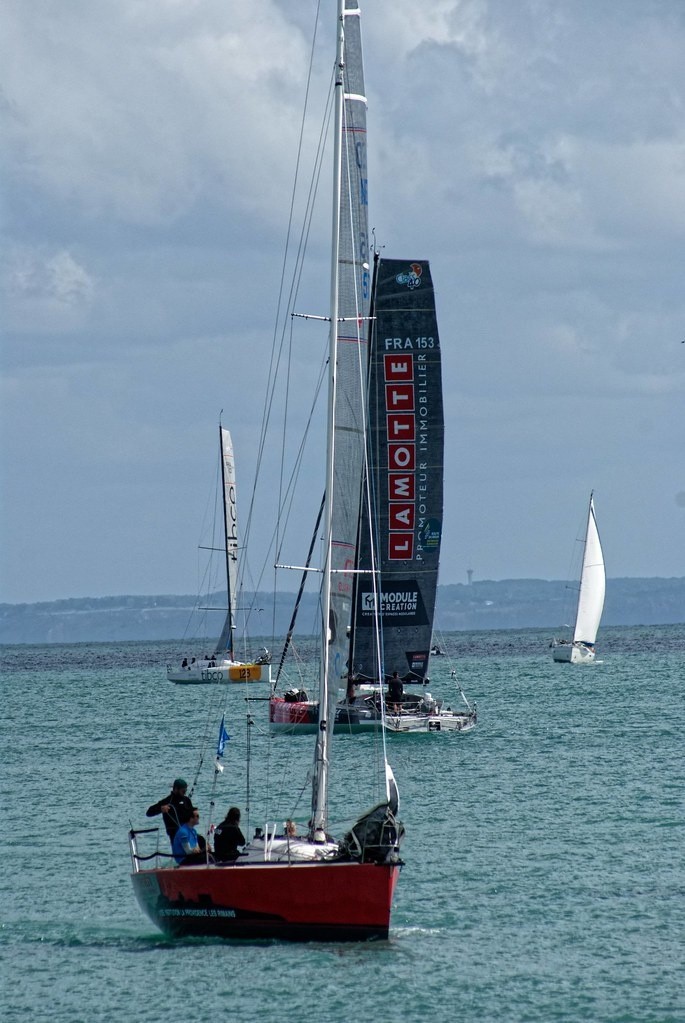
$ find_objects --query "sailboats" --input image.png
[128,0,405,943]
[268,0,478,733]
[166,426,273,684]
[549,493,606,664]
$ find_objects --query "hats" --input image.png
[173,779,187,788]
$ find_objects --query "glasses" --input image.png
[195,815,199,818]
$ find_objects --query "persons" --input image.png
[204,655,211,667]
[285,688,308,702]
[214,808,246,861]
[182,658,190,670]
[211,655,216,667]
[384,672,403,716]
[172,808,214,865]
[145,779,206,852]
[191,658,195,663]
